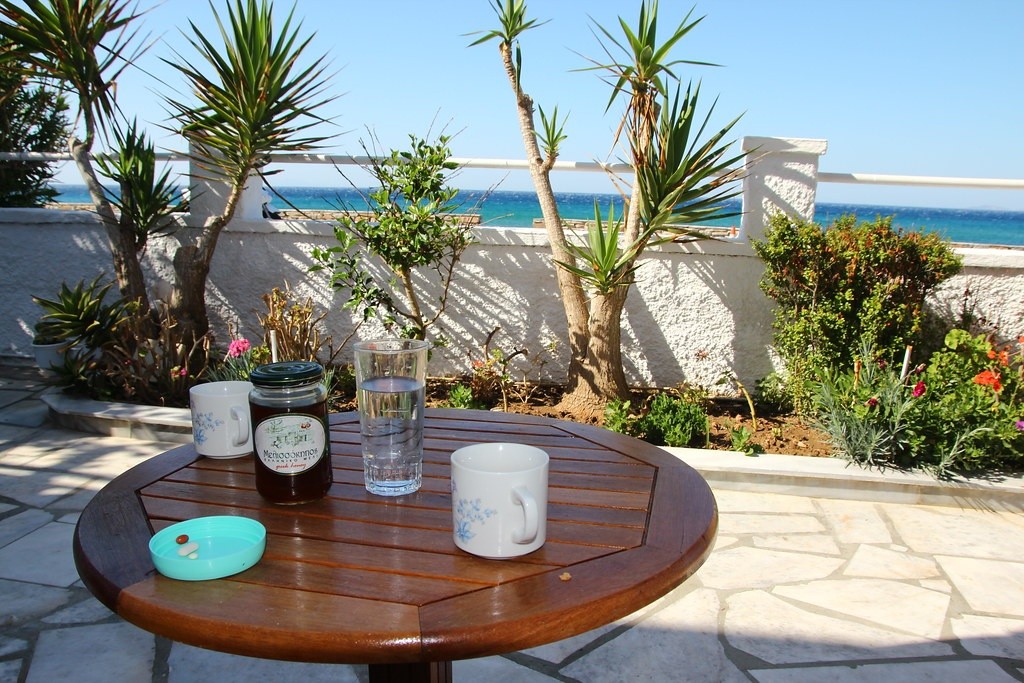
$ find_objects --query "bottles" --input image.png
[249,361,333,505]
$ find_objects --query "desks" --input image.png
[73,410,718,683]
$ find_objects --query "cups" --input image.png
[350,338,428,496]
[190,380,255,459]
[451,443,549,558]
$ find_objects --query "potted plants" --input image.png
[30,335,69,378]
